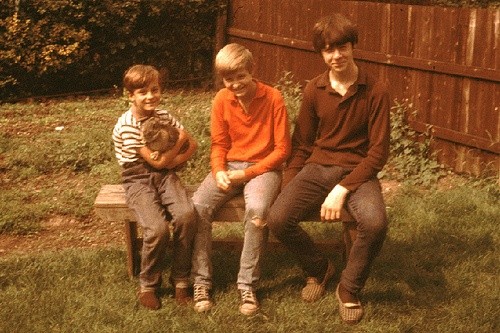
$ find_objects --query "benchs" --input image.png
[92,184,356,280]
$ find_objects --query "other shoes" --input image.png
[301,258,335,301]
[174,287,190,306]
[335,281,364,322]
[141,292,162,310]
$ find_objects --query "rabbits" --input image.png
[142,118,188,161]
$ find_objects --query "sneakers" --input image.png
[191,285,214,312]
[237,289,261,315]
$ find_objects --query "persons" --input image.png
[110,63,198,310]
[266,12,390,325]
[191,43,291,317]
